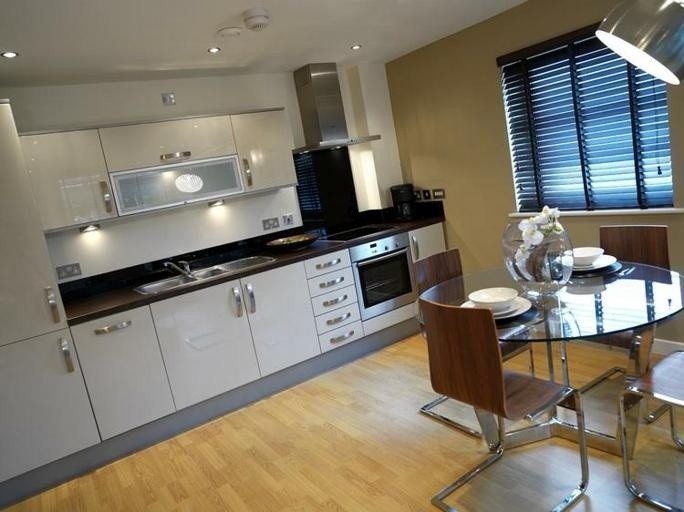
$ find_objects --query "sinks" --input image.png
[194,264,231,283]
[223,253,276,274]
[131,274,200,297]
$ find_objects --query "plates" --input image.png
[554,255,616,272]
[458,297,533,321]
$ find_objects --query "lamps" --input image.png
[595,0,683,87]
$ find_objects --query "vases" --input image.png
[501,222,575,297]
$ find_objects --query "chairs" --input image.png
[412,249,535,439]
[418,296,590,512]
[618,350,684,512]
[579,225,671,421]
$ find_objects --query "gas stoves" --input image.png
[317,218,400,242]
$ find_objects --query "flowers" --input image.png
[517,206,563,252]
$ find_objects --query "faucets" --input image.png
[160,258,191,278]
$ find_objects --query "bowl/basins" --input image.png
[563,247,604,266]
[467,287,517,309]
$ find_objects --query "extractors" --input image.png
[290,63,383,152]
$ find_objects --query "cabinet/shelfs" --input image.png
[304,248,365,352]
[0,328,102,486]
[19,130,118,232]
[231,106,298,199]
[0,98,68,346]
[150,261,321,414]
[409,222,447,263]
[70,303,177,441]
[97,115,236,175]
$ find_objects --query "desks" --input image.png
[415,259,684,460]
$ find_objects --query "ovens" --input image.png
[348,230,425,322]
